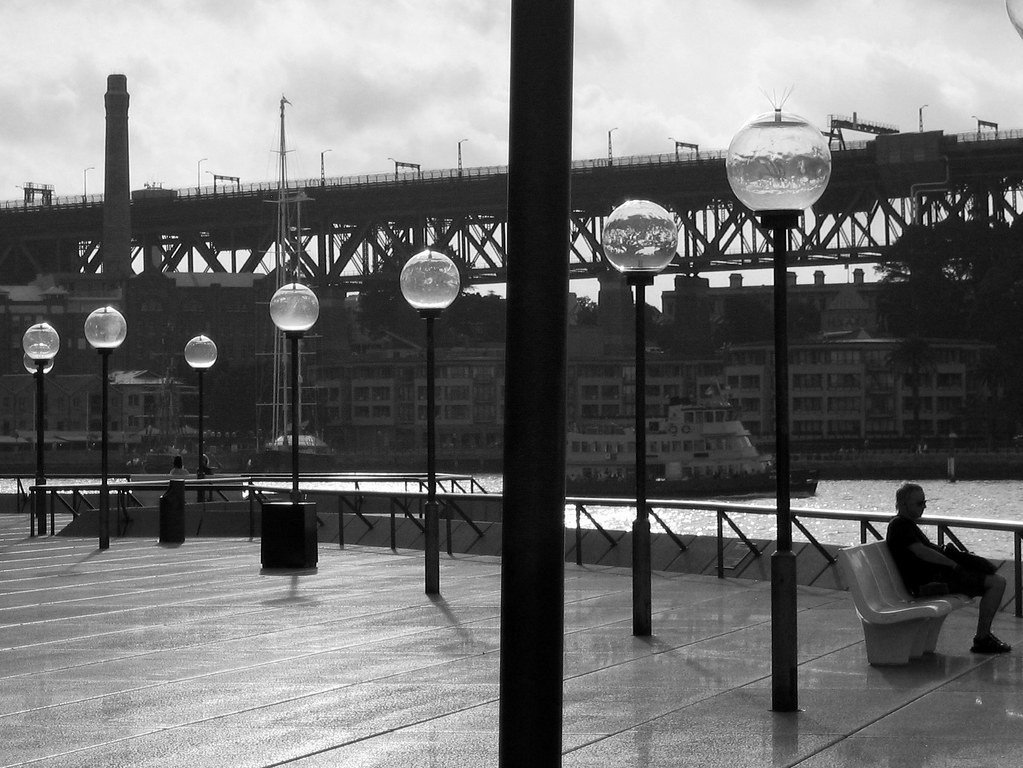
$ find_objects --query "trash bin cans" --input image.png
[159,480,186,544]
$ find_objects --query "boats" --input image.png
[564,375,824,501]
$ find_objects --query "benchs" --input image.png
[838,541,973,665]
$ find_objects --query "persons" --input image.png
[170,456,188,474]
[886,480,1011,652]
[198,455,215,474]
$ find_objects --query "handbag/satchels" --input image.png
[940,543,998,574]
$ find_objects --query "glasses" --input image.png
[908,500,926,508]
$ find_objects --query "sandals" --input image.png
[970,632,1012,653]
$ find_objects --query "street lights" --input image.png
[387,157,398,182]
[84,305,128,551]
[607,127,619,166]
[601,200,678,636]
[668,137,678,162]
[400,249,460,595]
[23,322,61,537]
[457,138,470,177]
[919,104,929,133]
[321,149,333,186]
[205,170,216,193]
[183,335,218,502]
[269,282,320,502]
[725,107,833,714]
[83,167,96,203]
[197,158,208,195]
[23,342,54,518]
[973,115,981,141]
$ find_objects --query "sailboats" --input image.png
[128,92,343,475]
